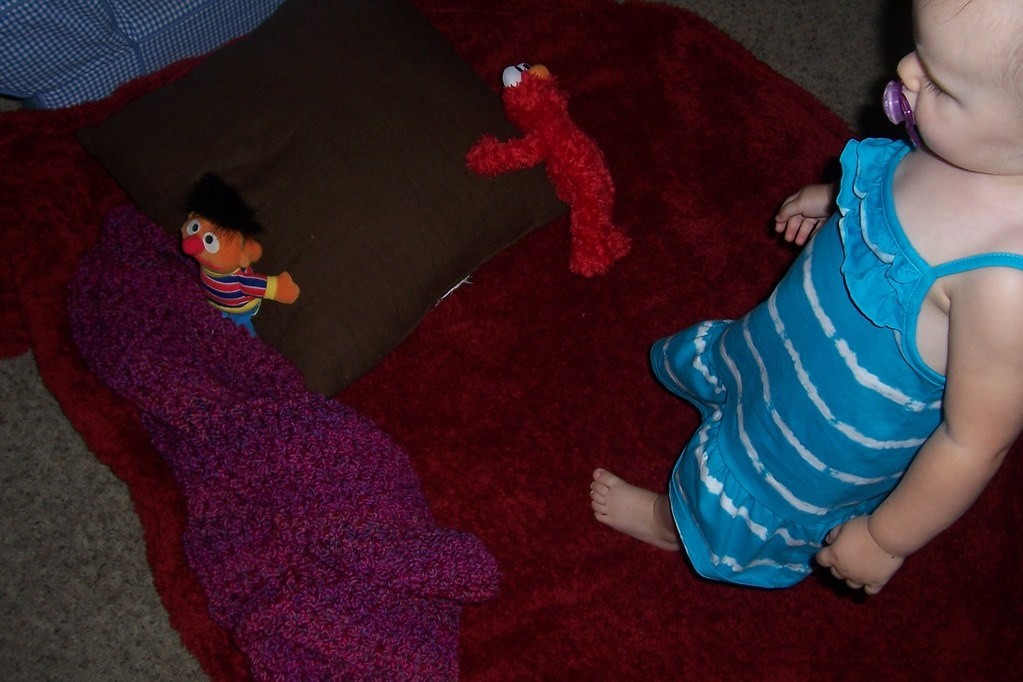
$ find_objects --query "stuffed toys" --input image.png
[465,62,631,278]
[180,194,301,337]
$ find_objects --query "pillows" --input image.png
[72,0,566,397]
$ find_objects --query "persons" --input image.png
[590,0,1023,596]
